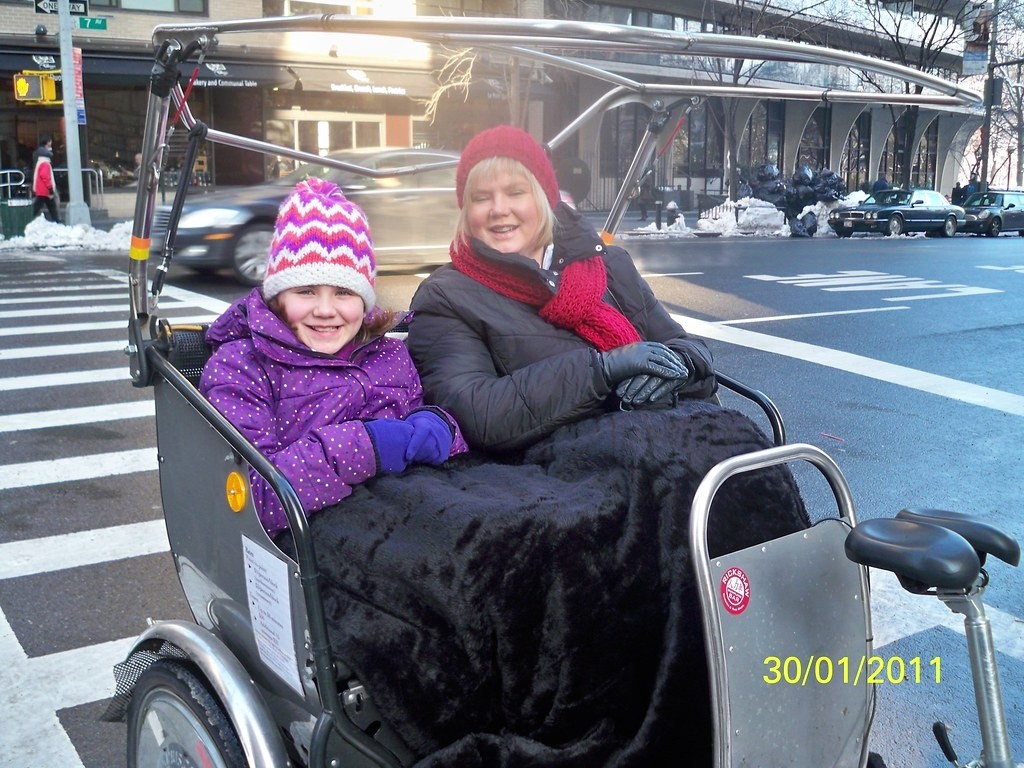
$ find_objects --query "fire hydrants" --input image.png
[666,200,682,227]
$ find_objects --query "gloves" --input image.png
[598,341,689,387]
[404,410,454,466]
[49,189,54,199]
[364,417,415,474]
[616,353,691,403]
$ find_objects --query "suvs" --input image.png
[957,191,1024,239]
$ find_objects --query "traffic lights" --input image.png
[13,74,56,102]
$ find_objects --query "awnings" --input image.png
[0,54,296,89]
[441,73,559,99]
[289,67,444,98]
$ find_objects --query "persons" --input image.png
[30,138,61,223]
[197,176,472,533]
[873,175,889,203]
[407,126,716,456]
[638,168,652,221]
[946,180,991,206]
[126,154,142,179]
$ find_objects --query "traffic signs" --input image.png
[33,0,90,17]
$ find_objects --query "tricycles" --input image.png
[97,14,1023,768]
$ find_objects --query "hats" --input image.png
[262,178,375,314]
[456,125,559,215]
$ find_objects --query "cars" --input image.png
[828,188,967,239]
[146,142,579,288]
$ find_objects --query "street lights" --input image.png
[1006,146,1016,191]
